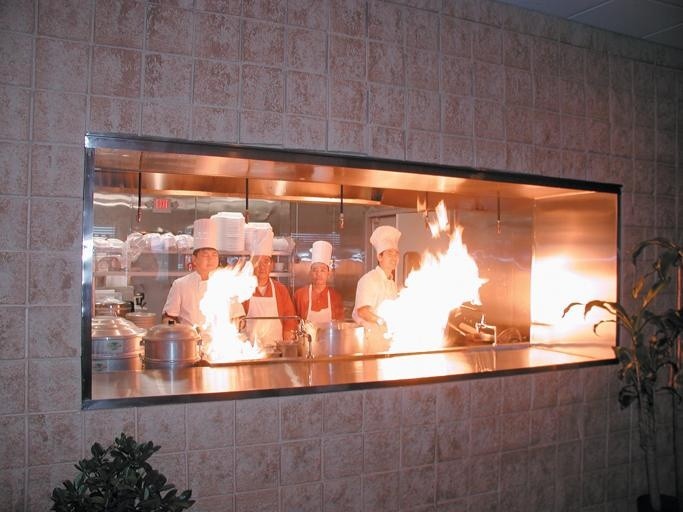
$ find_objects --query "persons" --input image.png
[162,218,221,330]
[351,225,402,330]
[294,241,344,324]
[243,225,299,342]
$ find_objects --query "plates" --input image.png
[126,311,155,328]
[212,210,275,256]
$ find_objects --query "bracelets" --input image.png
[376,318,384,326]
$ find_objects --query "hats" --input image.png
[246,227,274,257]
[370,225,402,254]
[192,218,218,253]
[310,240,333,267]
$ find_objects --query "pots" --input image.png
[89,316,200,371]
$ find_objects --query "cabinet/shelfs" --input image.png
[93,247,296,277]
